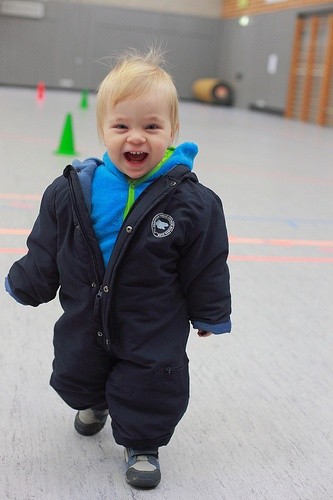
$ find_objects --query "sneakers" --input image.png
[123,447,161,489]
[74,408,109,436]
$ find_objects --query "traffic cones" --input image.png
[80,88,89,109]
[36,82,46,101]
[52,112,81,156]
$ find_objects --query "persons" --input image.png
[2,47,232,491]
